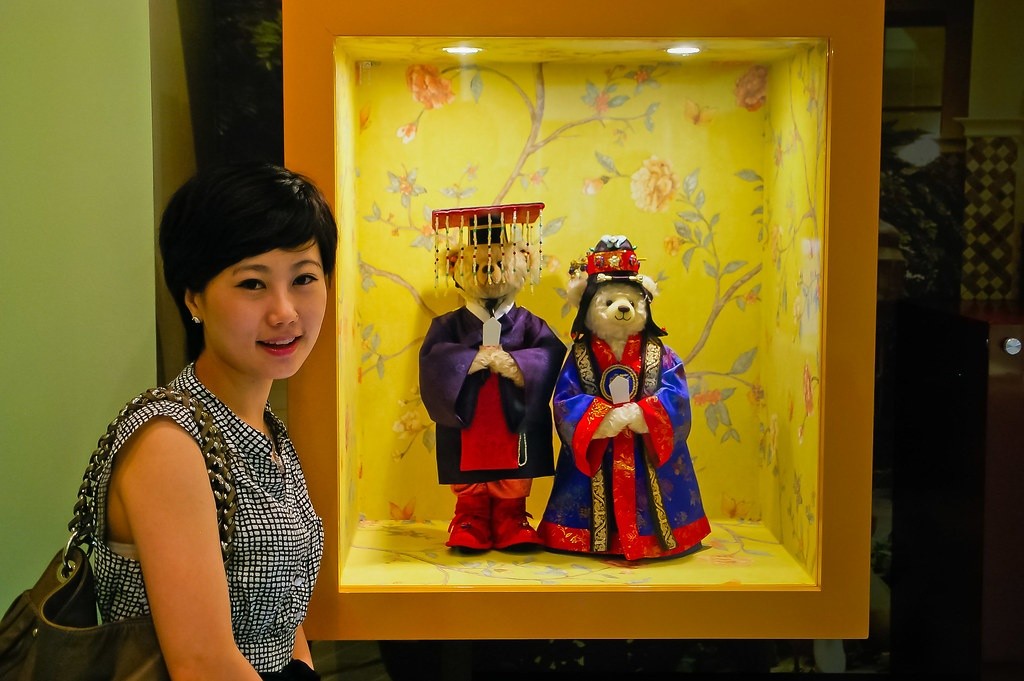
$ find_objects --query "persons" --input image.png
[89,161,339,681]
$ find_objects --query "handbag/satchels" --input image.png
[0,386,238,681]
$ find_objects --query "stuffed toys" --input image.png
[537,236,713,560]
[418,202,568,549]
